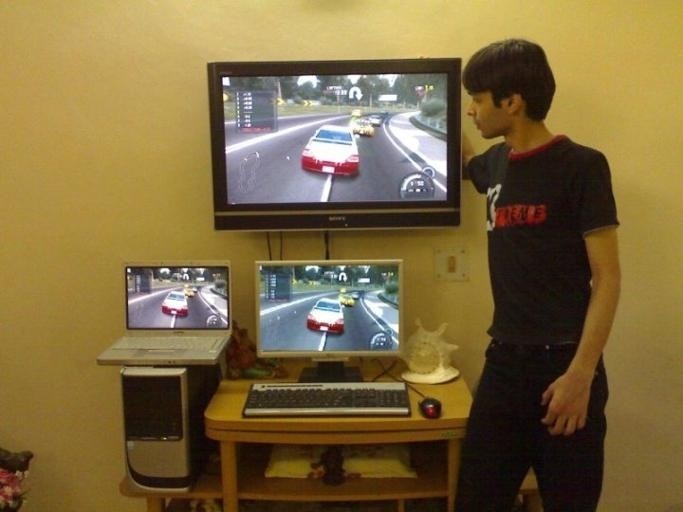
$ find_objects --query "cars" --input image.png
[306,297,345,333]
[337,294,354,308]
[161,290,187,317]
[340,288,360,300]
[351,108,389,127]
[350,119,374,137]
[300,123,359,178]
[183,283,197,297]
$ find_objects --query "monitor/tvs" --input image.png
[254,258,404,383]
[208,57,461,231]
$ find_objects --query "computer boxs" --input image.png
[120,365,220,493]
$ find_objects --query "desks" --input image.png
[118,363,543,512]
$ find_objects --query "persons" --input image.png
[453,38,625,510]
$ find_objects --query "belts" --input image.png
[486,337,577,372]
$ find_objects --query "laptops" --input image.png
[97,259,233,365]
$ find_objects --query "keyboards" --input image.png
[242,383,410,418]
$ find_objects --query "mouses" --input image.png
[420,398,441,418]
